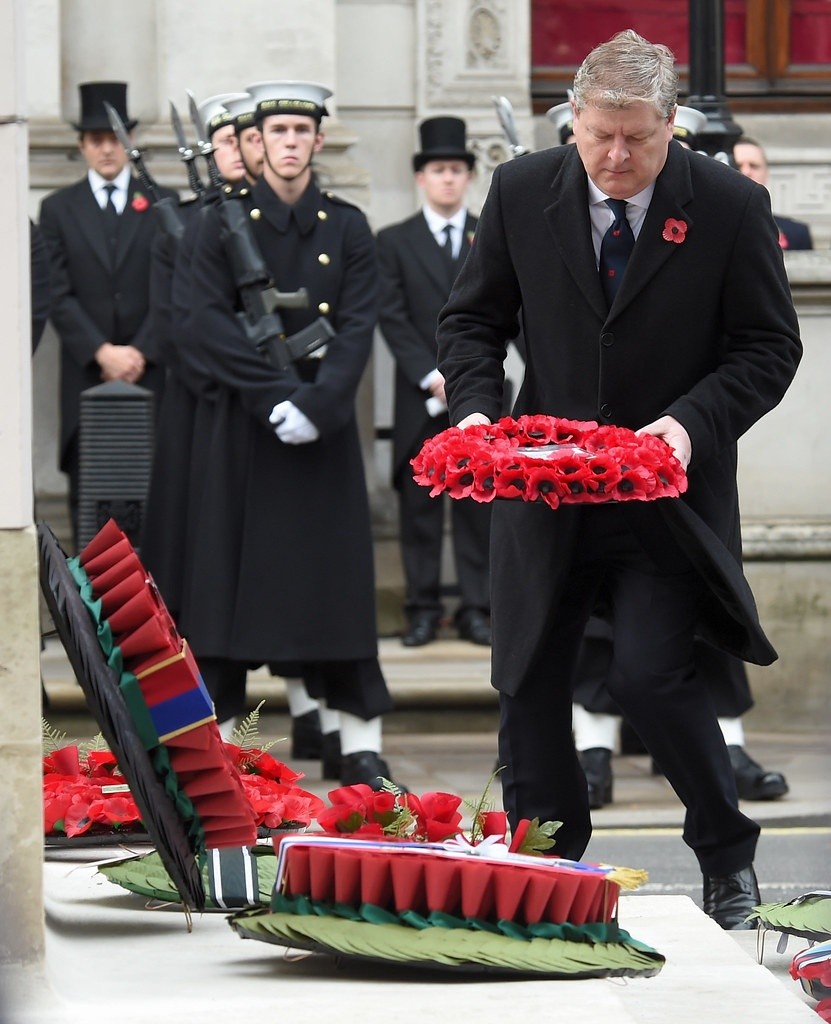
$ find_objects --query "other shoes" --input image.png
[341,752,408,792]
[322,731,341,778]
[293,708,324,757]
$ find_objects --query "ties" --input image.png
[598,198,636,311]
[102,185,120,265]
[443,224,454,258]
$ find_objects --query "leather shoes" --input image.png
[459,618,492,644]
[578,749,612,803]
[728,744,787,798]
[403,619,436,645]
[704,863,762,930]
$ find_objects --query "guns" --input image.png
[185,91,337,387]
[493,95,536,160]
[103,100,190,235]
[168,99,201,194]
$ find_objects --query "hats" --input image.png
[672,105,706,150]
[246,80,334,119]
[197,92,244,141]
[412,116,476,170]
[70,82,138,132]
[546,101,576,147]
[222,94,256,134]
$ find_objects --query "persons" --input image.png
[149,79,409,793]
[40,80,180,553]
[732,139,813,253]
[436,28,806,928]
[541,103,790,811]
[376,116,516,646]
[29,217,52,354]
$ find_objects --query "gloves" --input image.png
[269,400,319,445]
